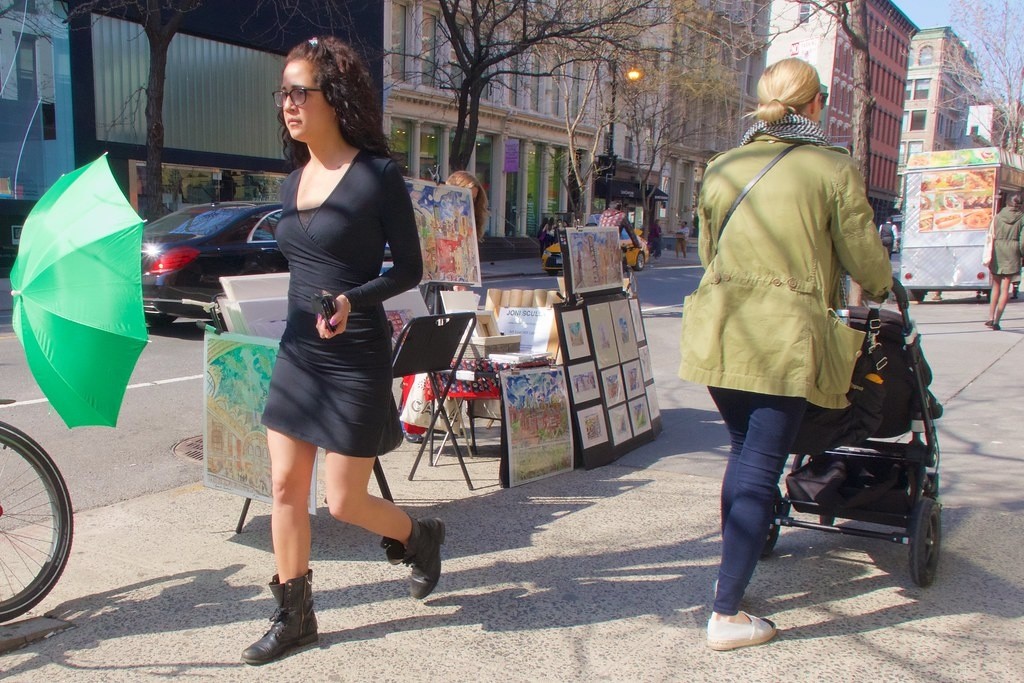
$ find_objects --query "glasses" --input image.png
[810,92,828,110]
[272,88,324,108]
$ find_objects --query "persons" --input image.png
[984,193,1024,331]
[675,221,688,258]
[597,200,640,298]
[400,171,487,444]
[879,216,897,260]
[537,217,569,258]
[241,36,447,666]
[676,59,893,652]
[653,219,662,258]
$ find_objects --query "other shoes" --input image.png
[993,324,1000,330]
[707,611,777,650]
[985,321,993,326]
[405,432,424,443]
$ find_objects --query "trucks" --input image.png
[899,146,1024,305]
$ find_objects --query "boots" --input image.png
[240,569,317,664]
[380,514,445,599]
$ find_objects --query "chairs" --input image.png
[324,310,476,504]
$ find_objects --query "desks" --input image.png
[426,357,554,466]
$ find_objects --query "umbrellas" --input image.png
[9,150,150,429]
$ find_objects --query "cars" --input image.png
[140,200,290,333]
[541,222,651,276]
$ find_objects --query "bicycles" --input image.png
[0,422,75,624]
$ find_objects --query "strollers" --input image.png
[760,269,944,589]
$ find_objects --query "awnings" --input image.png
[596,178,669,201]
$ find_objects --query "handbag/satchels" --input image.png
[982,216,994,267]
[789,308,888,457]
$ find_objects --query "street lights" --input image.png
[606,58,642,211]
